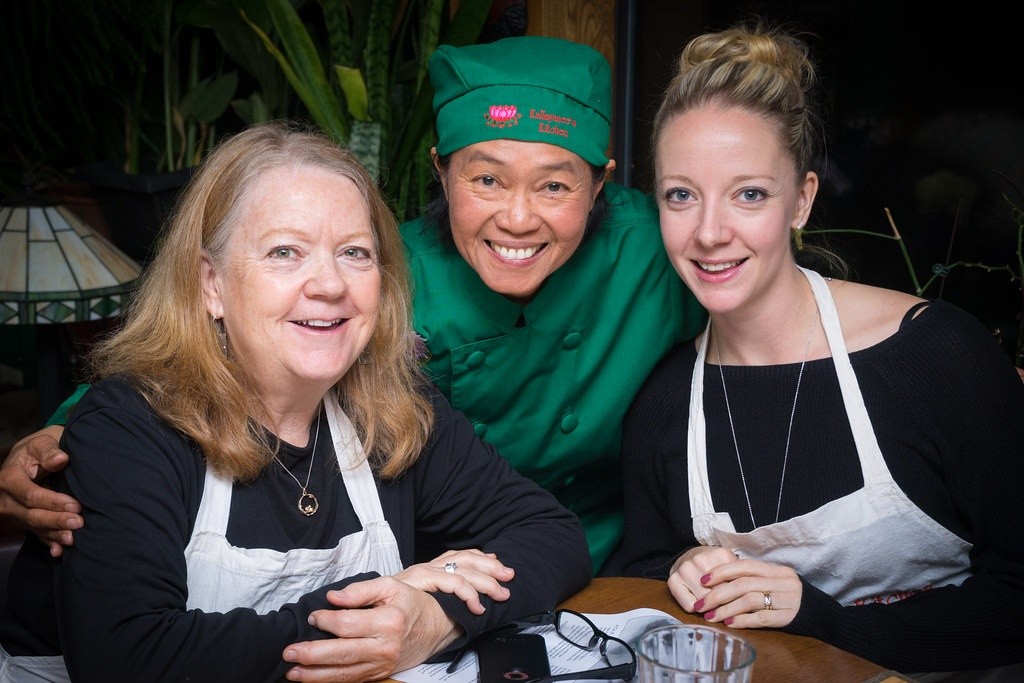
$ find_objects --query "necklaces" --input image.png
[712,276,832,530]
[247,401,322,516]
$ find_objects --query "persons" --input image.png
[599,30,1024,683]
[1,125,594,683]
[1,36,1024,683]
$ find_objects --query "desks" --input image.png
[373,576,906,683]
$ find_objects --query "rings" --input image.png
[445,563,458,573]
[763,591,773,610]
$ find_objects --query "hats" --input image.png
[426,36,614,166]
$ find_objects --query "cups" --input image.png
[637,624,756,683]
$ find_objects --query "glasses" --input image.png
[446,608,635,683]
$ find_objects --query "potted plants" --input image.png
[0,0,116,245]
[81,0,245,246]
